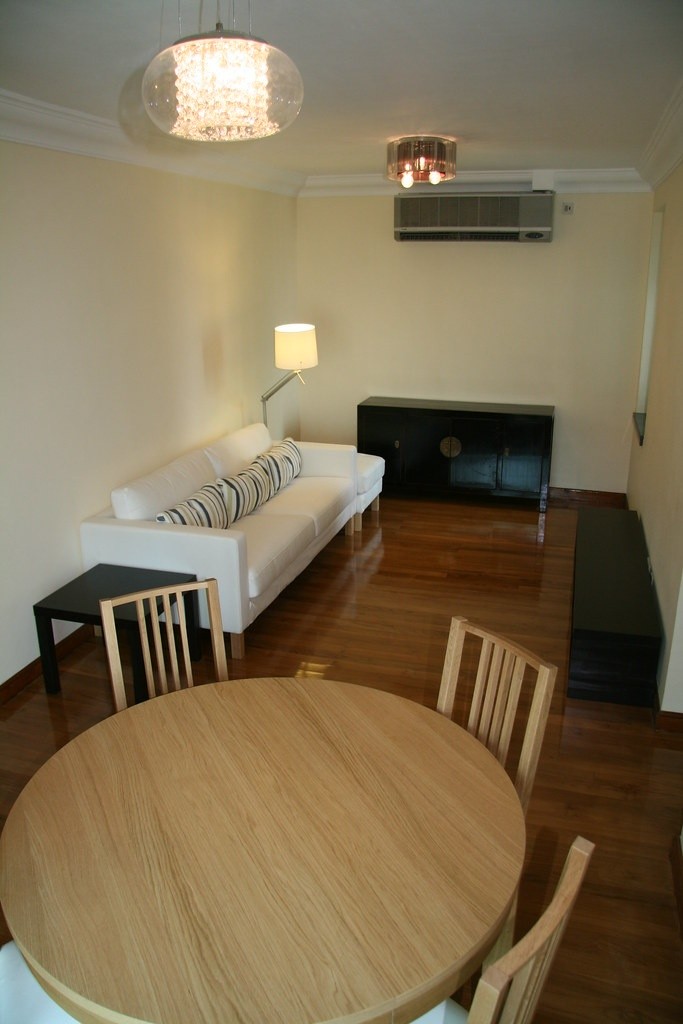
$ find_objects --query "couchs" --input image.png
[79,422,386,661]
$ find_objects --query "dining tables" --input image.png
[1,677,527,1024]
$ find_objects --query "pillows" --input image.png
[155,480,229,539]
[260,436,305,493]
[215,455,277,525]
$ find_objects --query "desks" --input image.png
[566,506,663,709]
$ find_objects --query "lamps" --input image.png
[384,135,457,189]
[139,1,303,142]
[260,322,319,426]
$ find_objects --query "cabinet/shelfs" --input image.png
[356,395,556,513]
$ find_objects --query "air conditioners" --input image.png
[394,189,554,243]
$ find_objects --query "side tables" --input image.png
[33,563,200,704]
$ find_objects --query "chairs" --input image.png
[1,938,88,1024]
[439,616,557,820]
[412,834,594,1024]
[98,576,228,712]
